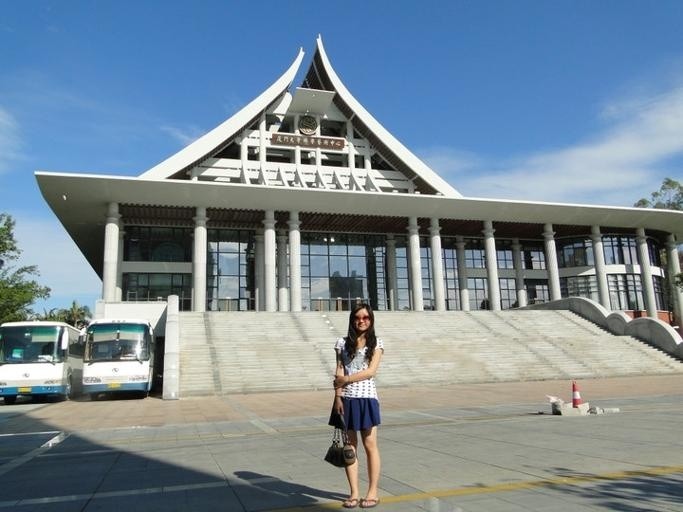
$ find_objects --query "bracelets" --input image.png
[334,394,342,398]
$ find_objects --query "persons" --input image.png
[328,302,386,508]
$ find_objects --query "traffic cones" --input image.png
[570,379,583,408]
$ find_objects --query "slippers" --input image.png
[361,497,379,508]
[344,498,358,508]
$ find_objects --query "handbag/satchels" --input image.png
[324,440,356,468]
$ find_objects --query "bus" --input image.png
[79,319,156,401]
[0,320,85,407]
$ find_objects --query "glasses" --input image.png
[353,315,370,323]
